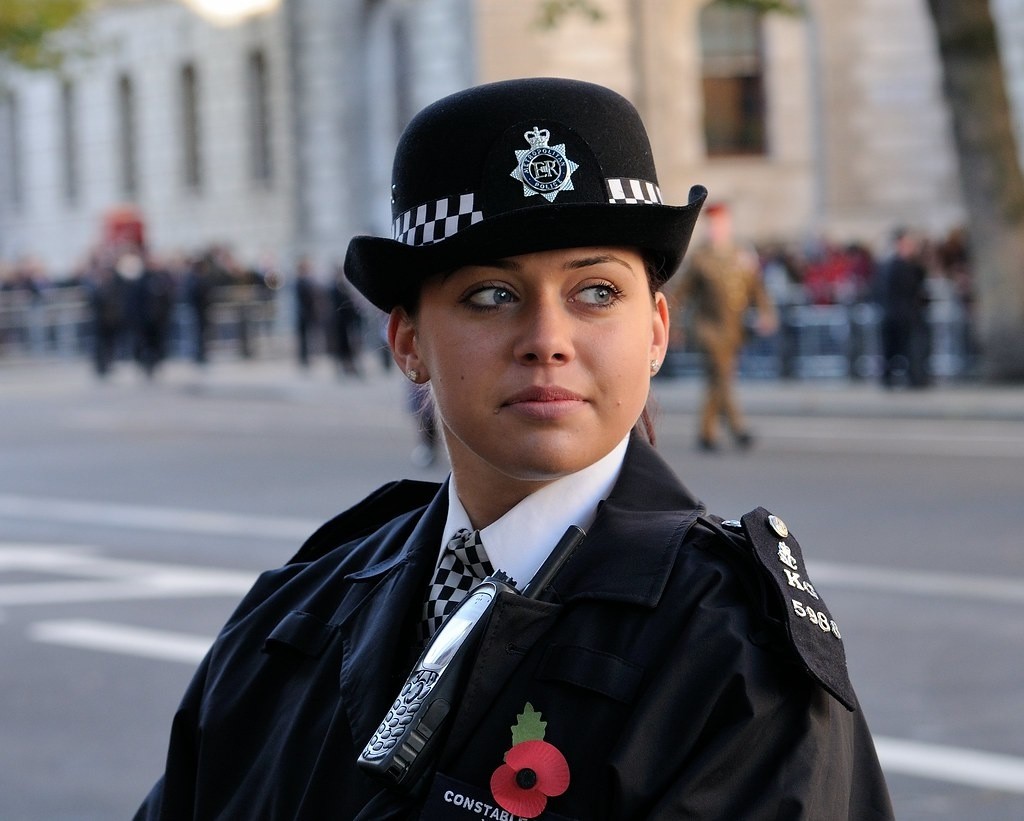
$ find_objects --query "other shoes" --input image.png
[697,432,753,453]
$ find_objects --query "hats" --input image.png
[342,78,709,312]
[706,202,726,214]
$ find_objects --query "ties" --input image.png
[426,528,495,637]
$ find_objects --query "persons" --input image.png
[130,77,892,821]
[672,203,780,453]
[0,226,977,390]
